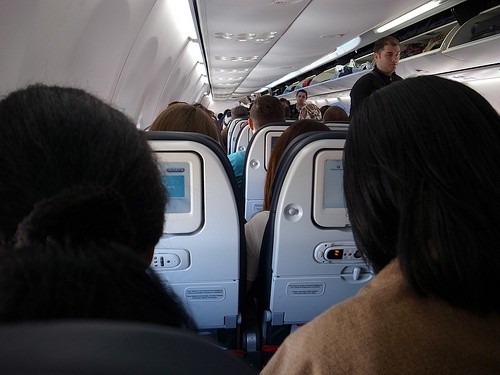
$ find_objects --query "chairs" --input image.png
[0,110,380,375]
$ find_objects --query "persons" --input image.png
[298,104,322,120]
[323,106,349,121]
[243,117,334,294]
[0,84,201,334]
[258,76,500,375]
[320,105,331,115]
[218,113,223,119]
[284,89,308,119]
[280,98,290,107]
[350,37,404,120]
[232,106,250,118]
[149,104,226,150]
[224,109,231,117]
